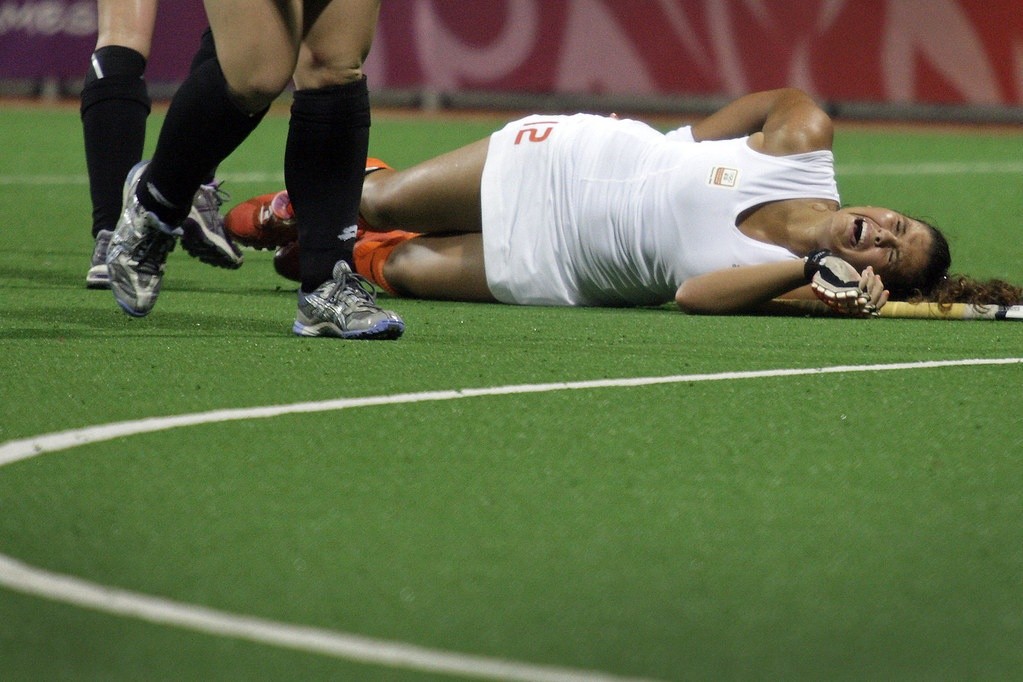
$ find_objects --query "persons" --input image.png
[220,90,952,318]
[78,0,245,286]
[104,0,407,340]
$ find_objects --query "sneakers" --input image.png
[224,159,399,284]
[86,160,244,316]
[291,261,404,338]
